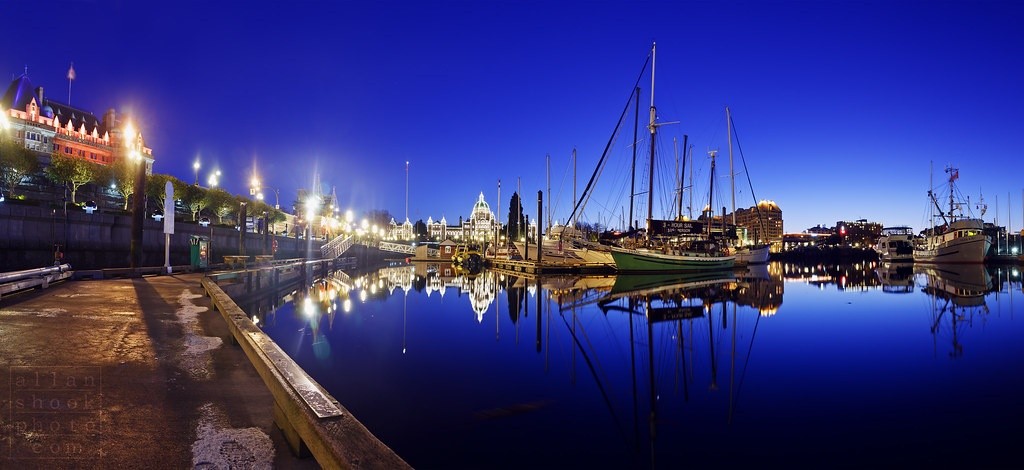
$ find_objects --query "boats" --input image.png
[910,161,992,263]
[876,224,913,263]
[910,263,997,356]
[875,261,915,294]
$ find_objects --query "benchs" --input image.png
[222,255,251,269]
[255,255,274,266]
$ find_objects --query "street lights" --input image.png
[193,164,387,241]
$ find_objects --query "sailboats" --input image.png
[480,43,775,305]
